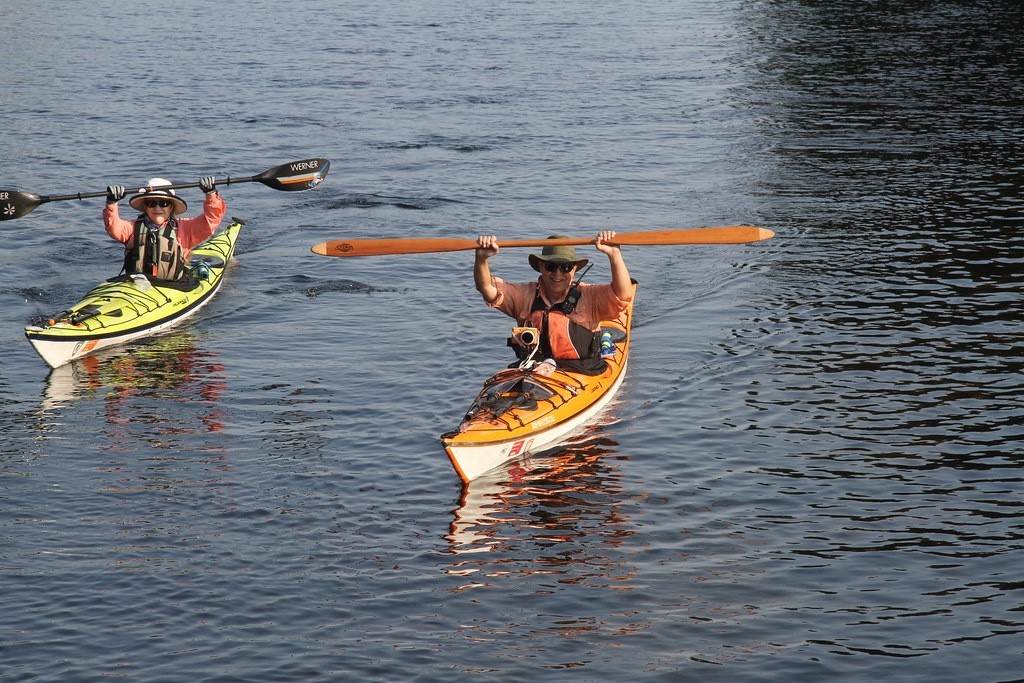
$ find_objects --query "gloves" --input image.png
[199,175,216,193]
[106,185,127,204]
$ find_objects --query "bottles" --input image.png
[601,333,611,355]
[197,260,209,281]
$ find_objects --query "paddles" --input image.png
[309,225,776,258]
[0,156,332,224]
[47,296,136,326]
[464,375,556,422]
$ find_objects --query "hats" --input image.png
[528,235,589,273]
[129,178,188,215]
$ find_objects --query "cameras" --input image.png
[511,327,539,345]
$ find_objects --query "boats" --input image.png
[441,276,637,482]
[24,215,246,368]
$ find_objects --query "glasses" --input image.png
[538,260,576,273]
[144,199,170,208]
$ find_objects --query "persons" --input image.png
[103,175,226,281]
[474,231,633,369]
